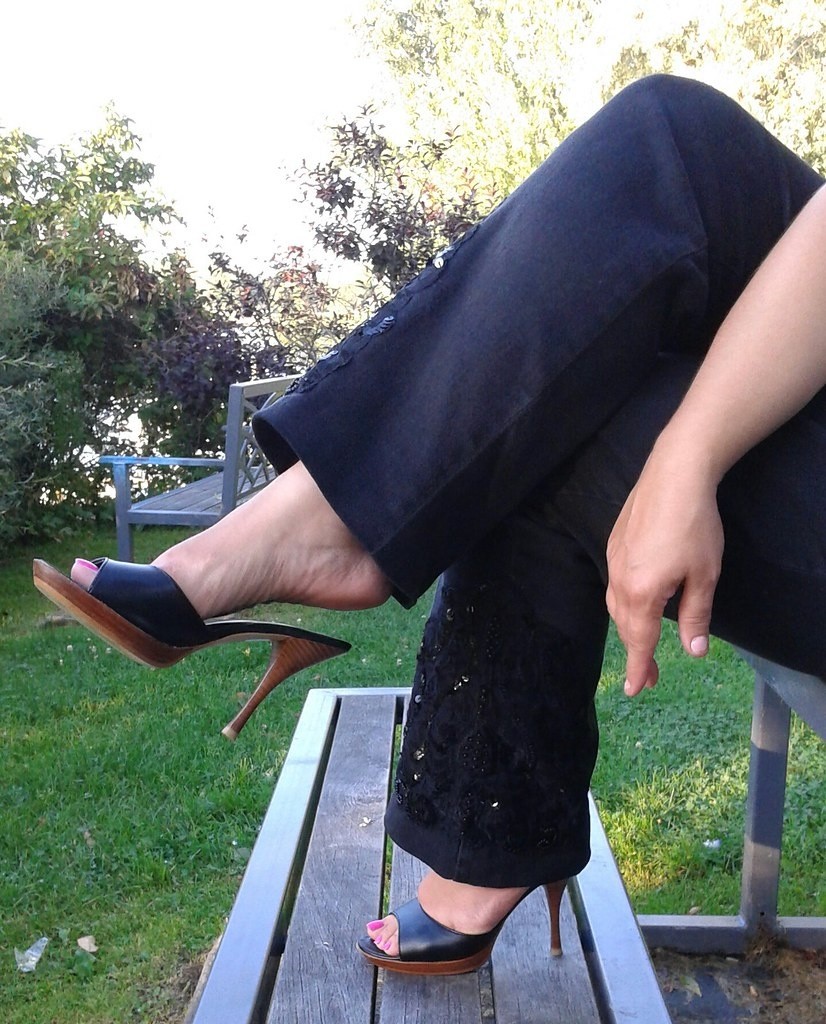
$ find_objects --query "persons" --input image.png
[30,74,826,976]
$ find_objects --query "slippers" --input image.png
[33,555,349,739]
[355,874,574,975]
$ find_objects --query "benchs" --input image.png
[98,374,304,562]
[182,688,674,1024]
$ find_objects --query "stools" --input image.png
[631,638,826,959]
